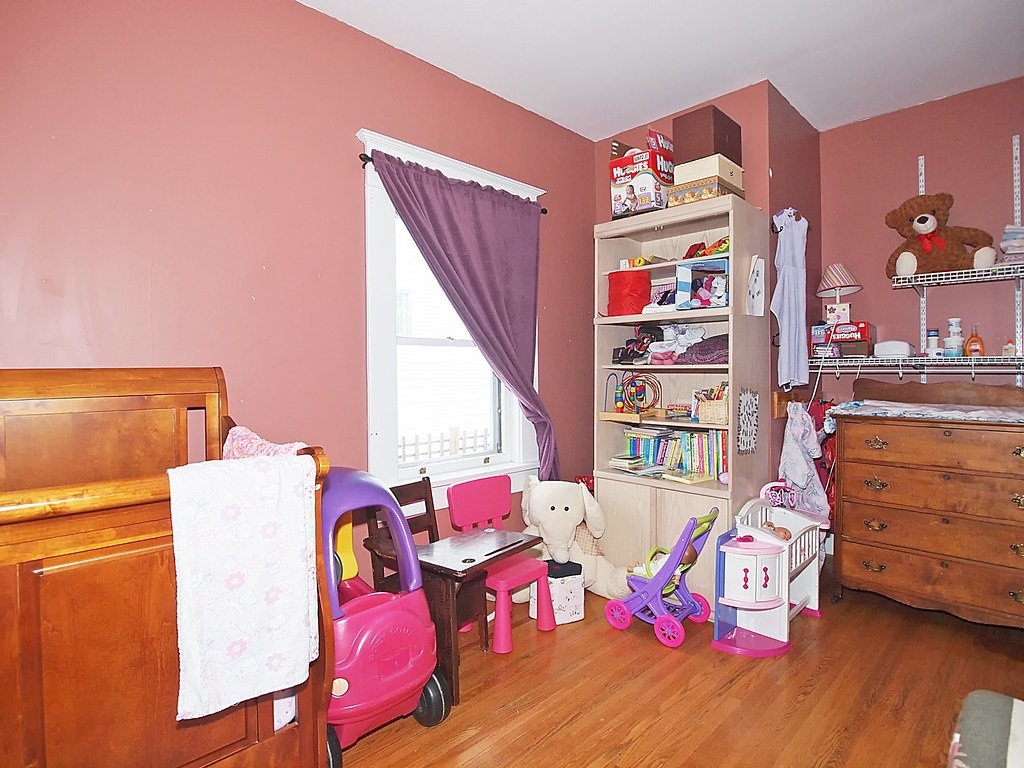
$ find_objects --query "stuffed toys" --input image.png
[885,192,997,279]
[512,474,638,606]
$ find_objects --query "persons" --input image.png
[628,544,697,585]
[655,182,661,191]
[754,520,792,542]
[613,185,639,212]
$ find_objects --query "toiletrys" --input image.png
[965,325,984,357]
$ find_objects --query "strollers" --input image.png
[603,506,719,648]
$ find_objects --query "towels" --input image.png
[166,454,319,721]
[1000,223,1024,262]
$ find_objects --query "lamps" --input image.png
[816,263,864,325]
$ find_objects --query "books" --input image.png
[667,379,729,420]
[609,426,730,484]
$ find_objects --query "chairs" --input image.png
[361,472,489,706]
[444,474,557,654]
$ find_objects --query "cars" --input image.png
[318,465,451,768]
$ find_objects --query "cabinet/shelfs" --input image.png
[718,539,784,609]
[591,473,771,627]
[803,262,1023,388]
[831,414,1023,630]
[594,196,770,499]
[15,522,271,768]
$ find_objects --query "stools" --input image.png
[529,559,586,626]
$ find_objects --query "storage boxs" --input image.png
[608,104,744,220]
[809,322,876,358]
